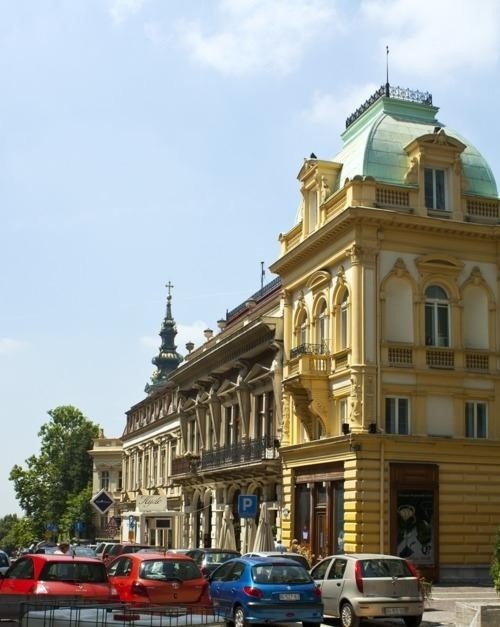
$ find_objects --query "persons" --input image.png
[275,540,287,552]
[49,543,71,578]
[35,545,46,554]
[273,536,277,546]
[291,539,300,553]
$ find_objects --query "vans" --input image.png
[307,552,424,626]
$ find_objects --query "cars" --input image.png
[203,556,324,626]
[0,552,120,619]
[105,552,215,617]
